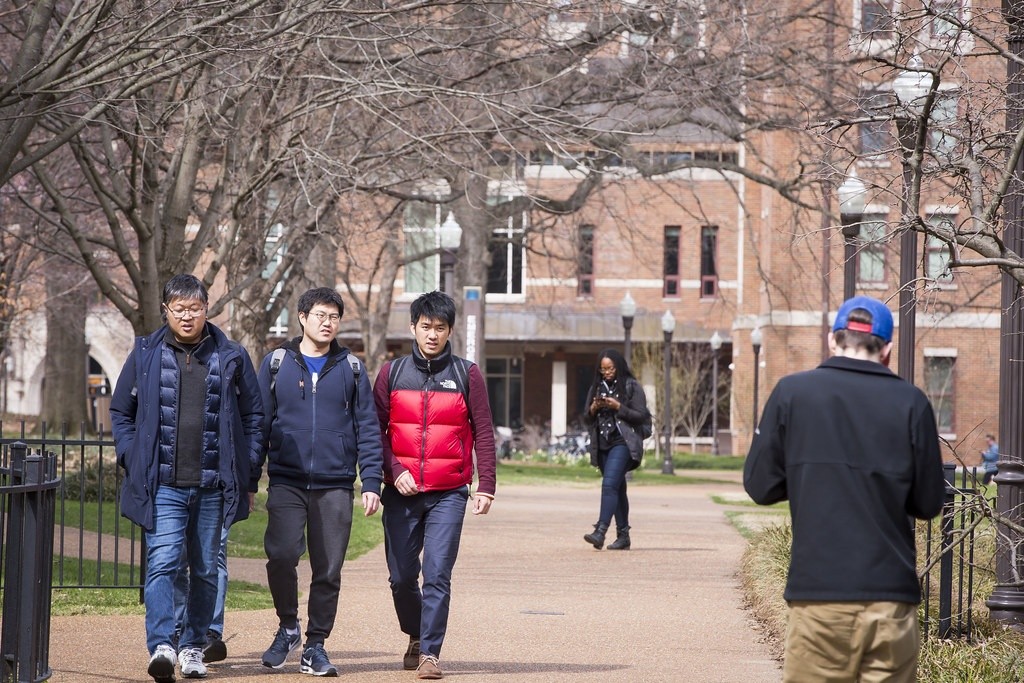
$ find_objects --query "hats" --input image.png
[831,296,894,367]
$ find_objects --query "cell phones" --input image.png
[594,395,605,403]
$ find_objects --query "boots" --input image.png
[606,523,632,550]
[583,520,610,549]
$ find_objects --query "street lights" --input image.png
[437,211,464,296]
[662,309,675,473]
[751,326,762,437]
[710,332,722,454]
[621,292,636,366]
[837,165,866,302]
[895,49,934,384]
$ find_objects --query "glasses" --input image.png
[308,311,343,323]
[166,303,208,319]
[597,366,619,374]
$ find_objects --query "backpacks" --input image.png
[624,376,653,439]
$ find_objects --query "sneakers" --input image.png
[261,622,302,670]
[401,634,423,670]
[147,644,178,683]
[417,652,444,679]
[177,645,209,680]
[300,641,339,677]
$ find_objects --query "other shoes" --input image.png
[200,630,228,663]
[171,627,181,654]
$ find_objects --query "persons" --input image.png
[979,434,999,485]
[109,273,265,683]
[373,291,496,679]
[583,349,646,551]
[744,297,946,683]
[248,288,383,678]
